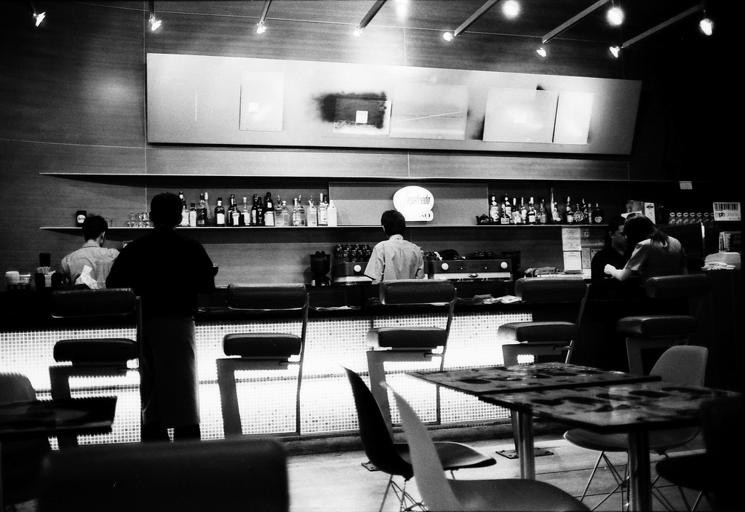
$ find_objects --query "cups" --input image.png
[126,208,153,228]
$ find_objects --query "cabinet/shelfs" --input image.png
[37,169,673,233]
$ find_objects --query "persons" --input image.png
[605,215,687,314]
[104,190,219,441]
[60,213,119,289]
[592,215,633,278]
[361,209,429,281]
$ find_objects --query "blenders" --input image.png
[310,250,331,287]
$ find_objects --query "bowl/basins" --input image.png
[5,271,19,285]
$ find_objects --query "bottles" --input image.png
[176,190,329,228]
[668,212,715,225]
[475,191,606,225]
